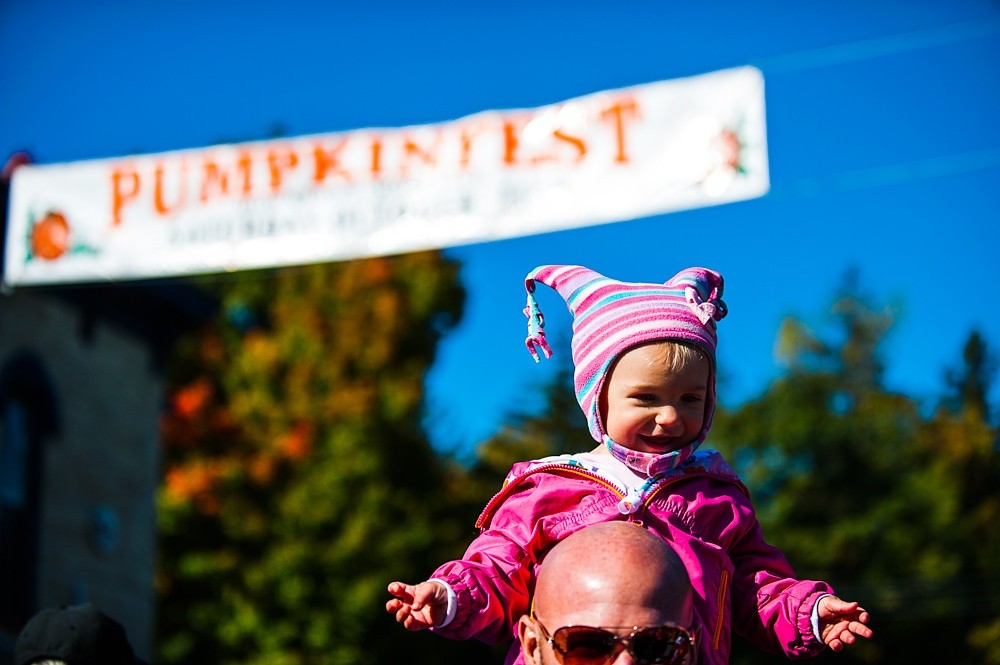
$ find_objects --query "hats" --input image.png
[523,264,730,479]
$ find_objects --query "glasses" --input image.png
[533,610,696,665]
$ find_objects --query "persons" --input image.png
[388,265,874,665]
[513,522,699,665]
